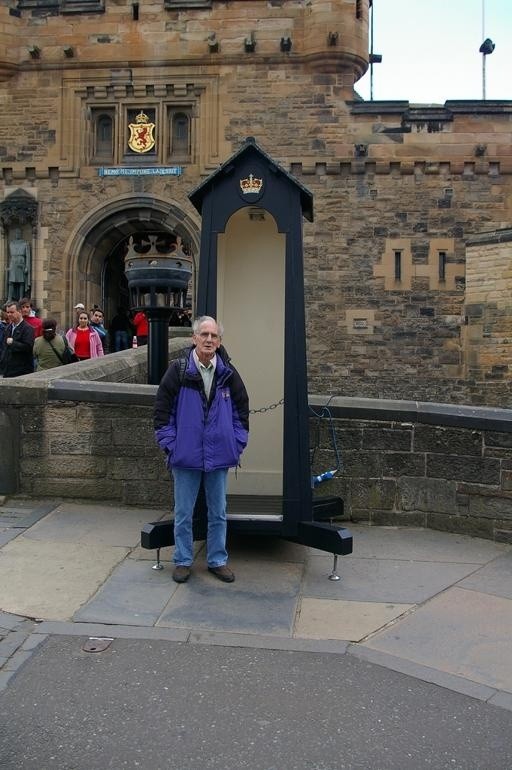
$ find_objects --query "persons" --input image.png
[154,315,249,583]
[0,294,148,379]
[168,308,192,327]
[6,227,31,303]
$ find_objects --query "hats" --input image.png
[74,303,84,309]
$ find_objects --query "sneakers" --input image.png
[208,565,234,583]
[173,566,191,582]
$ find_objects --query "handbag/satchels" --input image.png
[60,346,78,365]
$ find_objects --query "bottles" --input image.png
[132,336,137,349]
